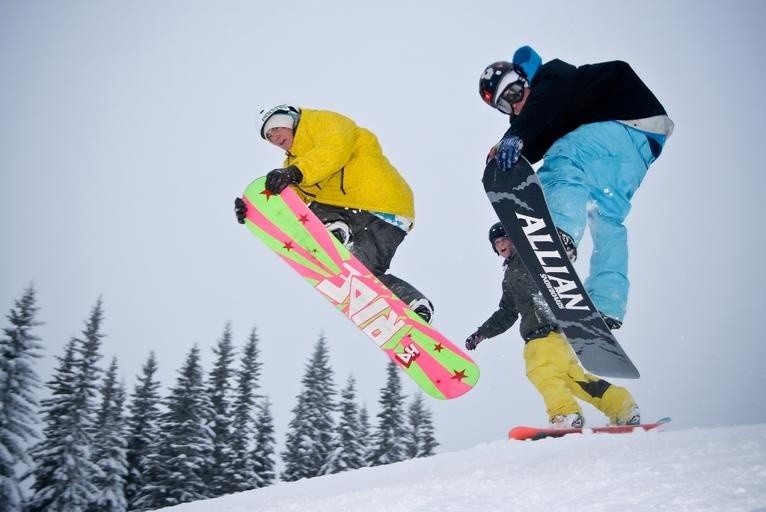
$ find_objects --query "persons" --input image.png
[478,47,671,328]
[234,105,435,325]
[466,222,639,427]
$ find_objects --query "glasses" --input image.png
[495,73,528,117]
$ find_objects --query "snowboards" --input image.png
[509,418,671,440]
[483,152,640,379]
[241,176,480,400]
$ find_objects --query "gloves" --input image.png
[466,332,484,348]
[234,198,248,224]
[495,135,523,171]
[265,165,302,194]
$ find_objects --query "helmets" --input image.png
[490,222,507,252]
[255,102,300,137]
[479,61,526,113]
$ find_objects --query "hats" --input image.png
[494,71,527,99]
[263,115,294,138]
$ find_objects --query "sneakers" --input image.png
[626,409,640,421]
[557,228,577,262]
[553,415,578,428]
[408,297,432,322]
[325,220,350,244]
